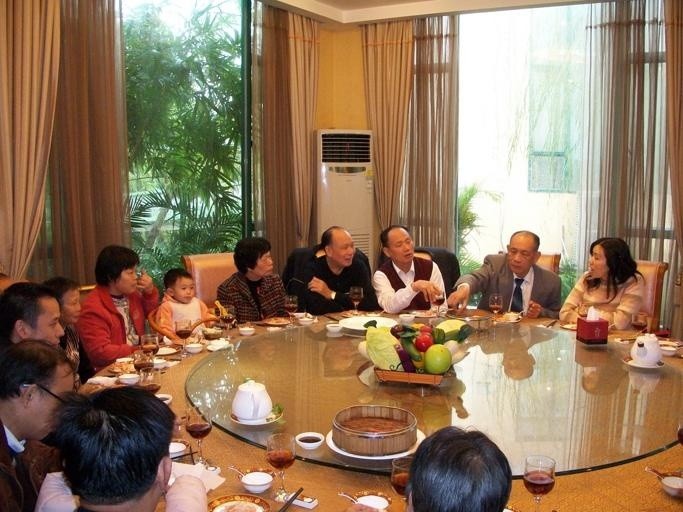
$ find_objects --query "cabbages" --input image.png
[436,319,466,332]
[366,325,405,371]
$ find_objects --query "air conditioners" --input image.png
[314,127,380,274]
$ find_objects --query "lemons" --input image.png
[412,351,425,368]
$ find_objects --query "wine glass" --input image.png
[432,291,447,317]
[523,455,557,512]
[490,294,504,323]
[285,297,299,329]
[347,286,364,316]
[265,432,297,504]
[174,319,191,347]
[184,408,215,474]
[218,305,236,339]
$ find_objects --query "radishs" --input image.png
[444,340,469,365]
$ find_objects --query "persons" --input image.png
[305,327,366,374]
[558,237,648,330]
[479,323,559,380]
[448,231,562,319]
[372,225,449,315]
[580,350,630,396]
[297,226,378,315]
[406,426,513,512]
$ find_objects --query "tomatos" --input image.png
[415,326,433,351]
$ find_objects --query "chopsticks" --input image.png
[327,313,340,323]
[277,487,303,511]
[546,320,557,328]
[170,445,198,466]
[256,321,285,330]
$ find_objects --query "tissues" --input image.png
[577,306,608,345]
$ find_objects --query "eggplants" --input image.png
[394,344,415,372]
[423,344,451,374]
[391,324,409,338]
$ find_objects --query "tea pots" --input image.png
[631,333,664,365]
[231,377,272,421]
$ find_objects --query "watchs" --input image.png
[331,291,336,300]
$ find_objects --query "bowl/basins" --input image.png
[352,489,392,511]
[506,314,519,320]
[240,471,272,494]
[656,472,683,495]
[660,345,677,359]
[113,354,138,386]
[186,319,340,358]
[167,442,186,456]
[399,315,416,322]
[296,432,325,451]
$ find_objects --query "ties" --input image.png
[511,278,524,313]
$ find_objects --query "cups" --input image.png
[133,333,167,396]
[390,456,415,497]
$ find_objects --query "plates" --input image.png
[411,310,435,318]
[155,394,172,405]
[207,494,271,512]
[507,320,520,323]
[339,317,398,331]
[324,428,426,462]
[622,356,664,371]
[560,320,578,330]
[158,344,184,355]
[228,408,284,425]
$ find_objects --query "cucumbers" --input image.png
[400,338,422,361]
[431,325,472,344]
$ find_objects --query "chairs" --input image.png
[279,243,371,310]
[496,251,561,276]
[180,251,239,318]
[626,259,670,335]
[376,246,461,302]
